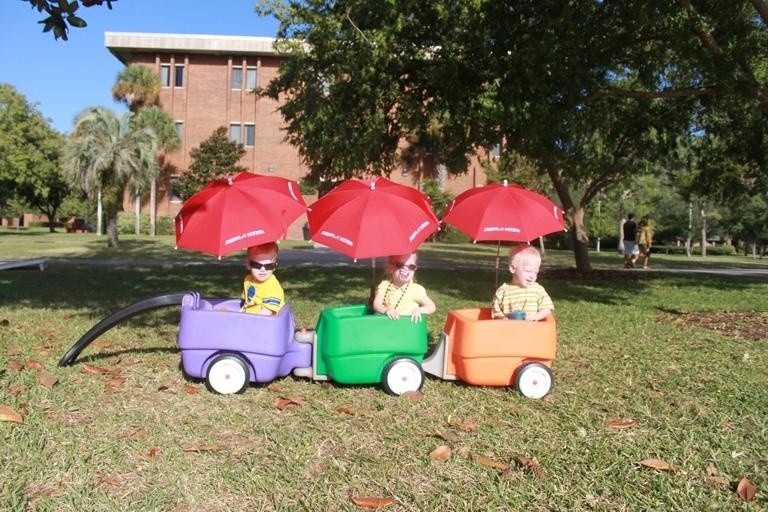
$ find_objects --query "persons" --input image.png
[618,214,639,264]
[636,213,653,269]
[240,242,284,317]
[370,251,436,325]
[622,212,640,268]
[487,244,554,322]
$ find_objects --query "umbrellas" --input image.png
[439,177,570,248]
[172,171,313,262]
[304,176,442,266]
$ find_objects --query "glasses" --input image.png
[248,259,278,272]
[392,262,418,271]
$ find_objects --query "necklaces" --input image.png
[382,280,411,309]
